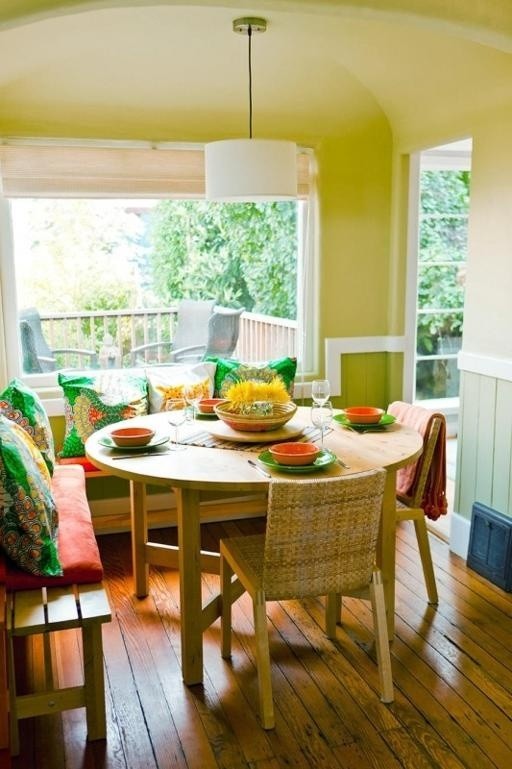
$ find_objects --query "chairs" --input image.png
[131,301,216,368]
[177,308,245,360]
[21,320,42,373]
[19,307,97,372]
[386,400,442,604]
[219,468,399,728]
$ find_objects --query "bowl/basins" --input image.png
[197,398,226,414]
[108,427,157,447]
[268,442,320,466]
[213,399,297,430]
[343,406,385,424]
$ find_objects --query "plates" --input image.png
[257,448,337,473]
[332,413,396,429]
[97,432,171,452]
[185,405,220,419]
[208,419,306,443]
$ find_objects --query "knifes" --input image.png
[112,453,170,460]
[247,460,271,478]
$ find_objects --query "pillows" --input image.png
[57,371,149,458]
[204,355,296,400]
[1,416,62,577]
[147,362,216,413]
[0,378,54,476]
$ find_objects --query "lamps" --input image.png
[204,139,297,203]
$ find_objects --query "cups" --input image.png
[311,380,331,434]
[184,380,204,426]
[165,398,188,451]
[311,400,333,453]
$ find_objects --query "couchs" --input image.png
[0,464,110,755]
[46,415,269,535]
